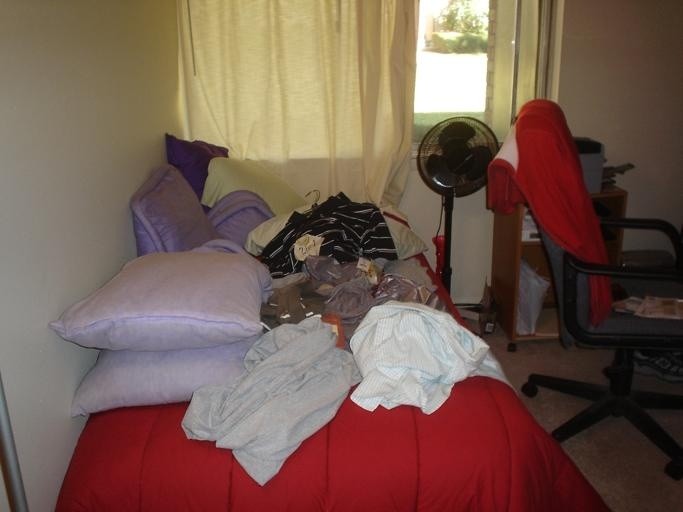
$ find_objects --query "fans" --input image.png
[416,116,500,298]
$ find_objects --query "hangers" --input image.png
[301,189,320,216]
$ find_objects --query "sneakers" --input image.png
[634,349,683,382]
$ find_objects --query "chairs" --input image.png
[515,100,681,481]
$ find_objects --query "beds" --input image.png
[55,206,540,512]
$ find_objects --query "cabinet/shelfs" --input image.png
[488,186,627,352]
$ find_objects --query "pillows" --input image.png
[47,134,308,418]
[244,201,429,262]
